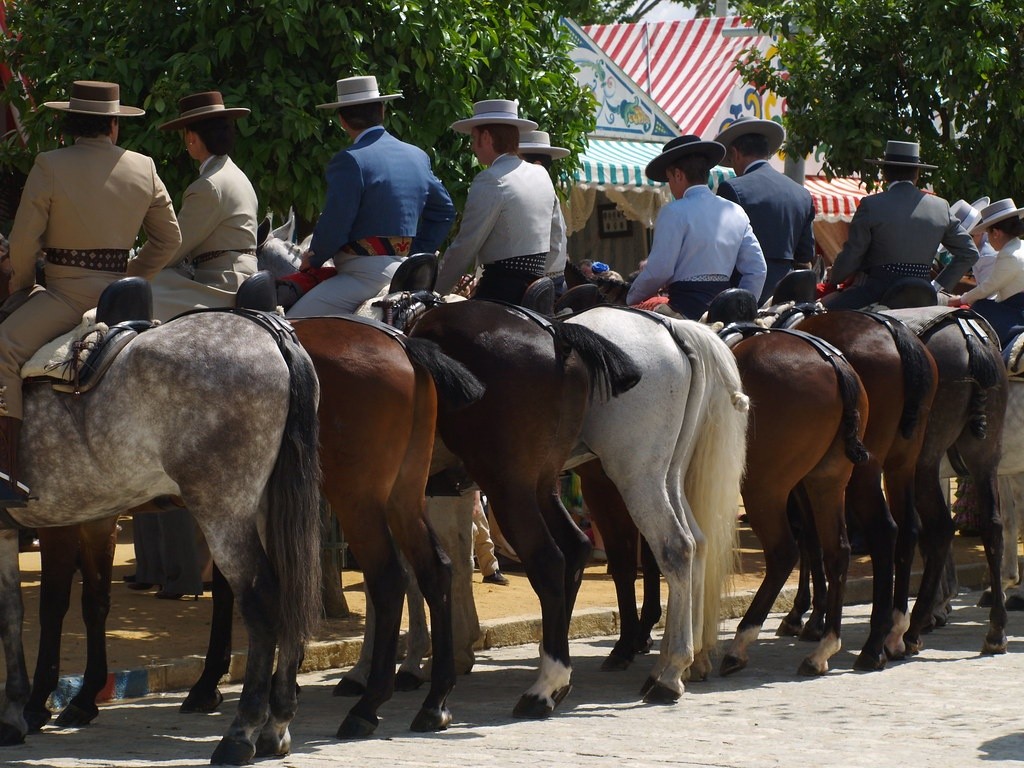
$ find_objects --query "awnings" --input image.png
[803,174,885,224]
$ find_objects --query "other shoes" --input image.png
[123,575,136,582]
[960,530,980,537]
[482,572,508,585]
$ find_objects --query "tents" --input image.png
[549,18,806,281]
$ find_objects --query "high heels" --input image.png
[127,582,160,590]
[155,591,199,602]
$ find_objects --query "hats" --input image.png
[645,135,726,182]
[518,131,571,161]
[937,196,990,253]
[315,76,402,109]
[450,99,538,134]
[44,80,146,116]
[712,116,784,168]
[864,140,939,169]
[159,92,251,129]
[969,198,1024,235]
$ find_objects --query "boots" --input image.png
[0,416,23,500]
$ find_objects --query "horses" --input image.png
[1,277,321,764]
[333,303,751,705]
[574,330,870,676]
[180,252,642,721]
[53,270,486,742]
[639,312,938,673]
[776,305,1024,657]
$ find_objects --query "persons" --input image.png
[712,116,816,309]
[148,91,258,324]
[0,80,183,501]
[434,100,563,304]
[937,198,1024,350]
[516,131,567,296]
[284,75,455,318]
[937,196,999,315]
[626,134,767,323]
[471,490,510,587]
[817,140,980,310]
